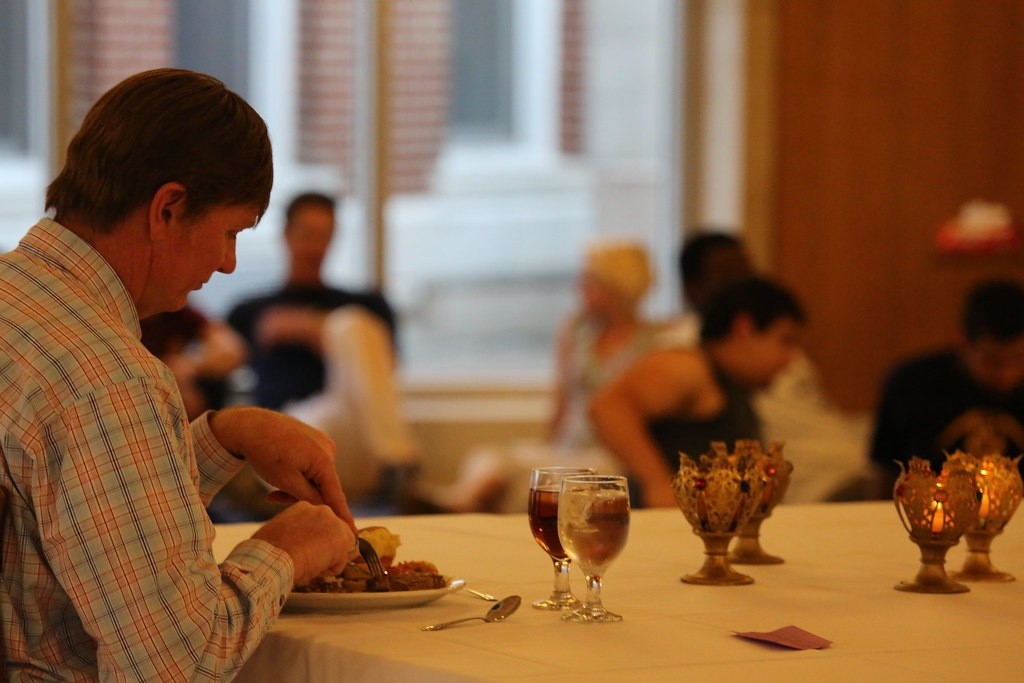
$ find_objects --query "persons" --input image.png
[136,192,1024,522]
[0,69,363,682]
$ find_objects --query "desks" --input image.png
[215,500,1024,683]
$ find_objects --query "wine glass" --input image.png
[556,476,631,622]
[527,468,597,609]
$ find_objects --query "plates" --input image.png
[285,580,466,612]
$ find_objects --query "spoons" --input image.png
[421,596,521,630]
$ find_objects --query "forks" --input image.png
[356,536,384,578]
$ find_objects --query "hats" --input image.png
[580,239,656,305]
